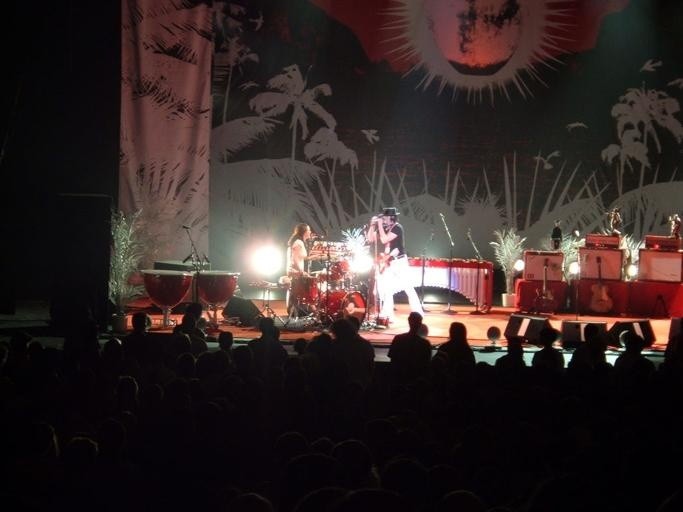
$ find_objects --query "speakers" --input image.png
[504,312,655,349]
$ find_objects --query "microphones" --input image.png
[182,225,191,230]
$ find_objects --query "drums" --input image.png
[141,270,192,330]
[291,276,316,304]
[321,292,365,327]
[190,271,240,325]
[319,261,348,281]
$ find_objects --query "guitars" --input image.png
[373,248,399,273]
[534,259,557,311]
[590,257,613,313]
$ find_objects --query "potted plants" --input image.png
[488,226,527,307]
[107,207,147,333]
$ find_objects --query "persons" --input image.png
[285,221,335,280]
[366,206,424,321]
[1,296,681,510]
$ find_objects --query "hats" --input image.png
[378,208,400,218]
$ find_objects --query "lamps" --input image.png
[503,312,656,350]
